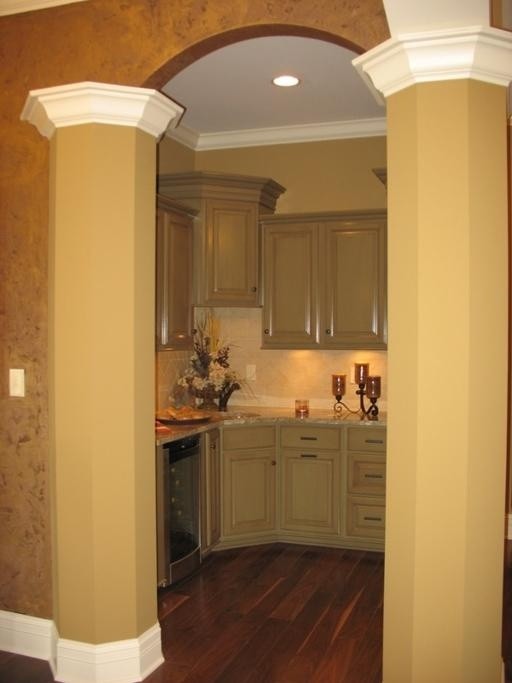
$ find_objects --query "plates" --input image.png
[156,407,214,426]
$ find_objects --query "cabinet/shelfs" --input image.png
[258,209,389,350]
[155,192,194,350]
[157,169,286,307]
[207,406,385,553]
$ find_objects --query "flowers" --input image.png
[175,309,246,388]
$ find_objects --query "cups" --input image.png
[294,398,310,422]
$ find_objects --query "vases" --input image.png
[194,388,219,408]
